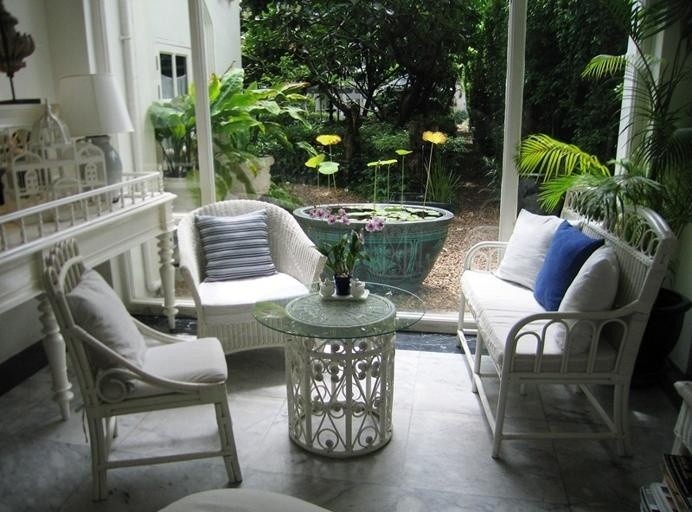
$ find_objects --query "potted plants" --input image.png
[514,1,690,386]
[147,60,321,213]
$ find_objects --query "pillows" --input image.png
[70,266,147,391]
[196,208,278,282]
[494,207,622,356]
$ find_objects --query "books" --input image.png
[639,454,692,511]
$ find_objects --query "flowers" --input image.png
[310,207,386,276]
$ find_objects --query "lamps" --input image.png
[56,70,136,206]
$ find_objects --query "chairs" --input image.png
[176,198,329,358]
[42,236,242,504]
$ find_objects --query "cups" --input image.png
[348,276,366,298]
[319,277,335,297]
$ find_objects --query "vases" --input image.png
[333,275,352,296]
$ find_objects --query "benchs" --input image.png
[458,187,679,460]
